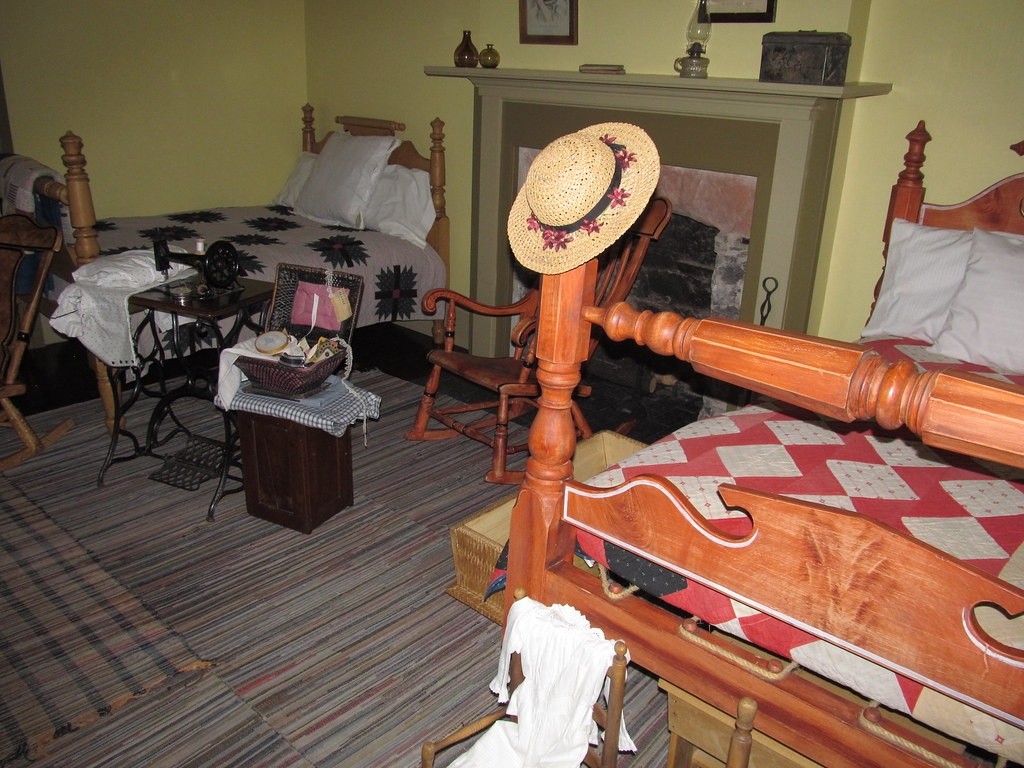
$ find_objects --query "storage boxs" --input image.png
[449,422,667,614]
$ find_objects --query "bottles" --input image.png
[479,44,500,68]
[454,30,479,67]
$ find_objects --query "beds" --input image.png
[494,120,1023,768]
[67,104,446,351]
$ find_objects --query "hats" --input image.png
[507,122,661,275]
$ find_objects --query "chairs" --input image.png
[421,588,628,768]
[1,212,77,476]
[417,203,668,492]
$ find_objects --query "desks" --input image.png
[237,380,354,534]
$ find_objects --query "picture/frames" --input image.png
[695,0,777,24]
[518,1,579,45]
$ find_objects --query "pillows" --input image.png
[292,133,402,230]
[924,229,1024,378]
[860,217,1024,348]
[285,150,442,247]
